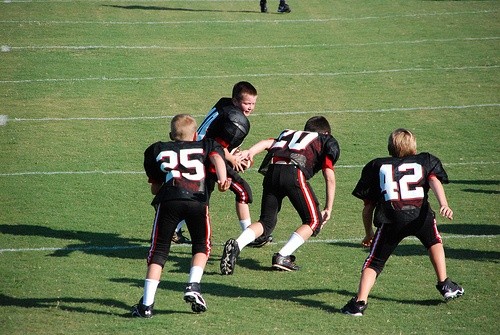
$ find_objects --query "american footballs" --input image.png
[230,150,254,171]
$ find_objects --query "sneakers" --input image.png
[184,282,207,312]
[340,295,368,315]
[122,297,155,318]
[272,253,298,271]
[260,1,267,12]
[277,3,291,12]
[435,281,465,299]
[169,228,192,246]
[220,238,240,274]
[247,235,273,247]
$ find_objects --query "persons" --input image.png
[172,81,272,250]
[129,114,231,318]
[340,128,464,316]
[260,0,291,14]
[220,116,340,276]
